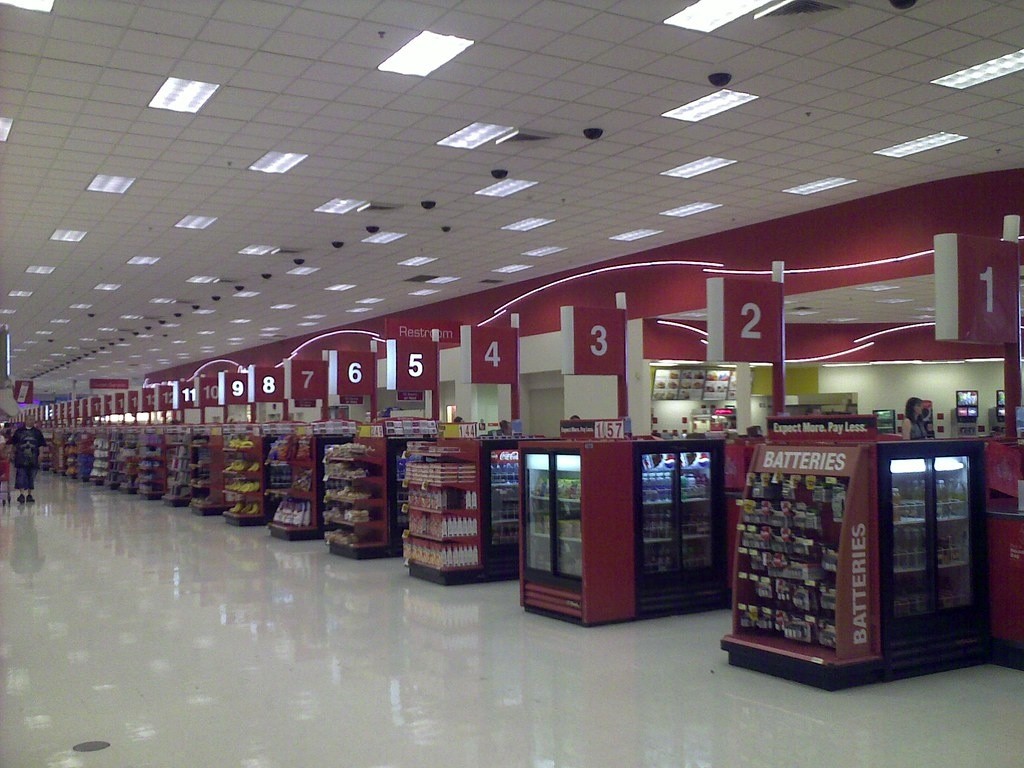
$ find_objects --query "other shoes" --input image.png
[26,495,34,503]
[17,494,25,503]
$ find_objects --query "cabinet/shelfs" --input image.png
[191,435,225,516]
[222,437,264,526]
[268,436,318,541]
[43,428,192,508]
[402,437,484,587]
[327,423,390,559]
[719,444,876,692]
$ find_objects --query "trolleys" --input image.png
[0,444,13,507]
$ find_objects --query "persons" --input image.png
[901,397,935,440]
[805,405,821,415]
[0,415,51,503]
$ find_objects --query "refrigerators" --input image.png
[876,439,990,677]
[638,437,728,616]
[517,440,635,624]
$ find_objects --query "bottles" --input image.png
[492,524,518,546]
[491,499,519,520]
[893,524,959,569]
[466,490,477,509]
[892,477,965,524]
[491,462,519,483]
[397,458,407,478]
[642,472,708,503]
[642,508,709,540]
[269,469,291,487]
[442,517,477,537]
[642,542,710,572]
[442,544,478,566]
